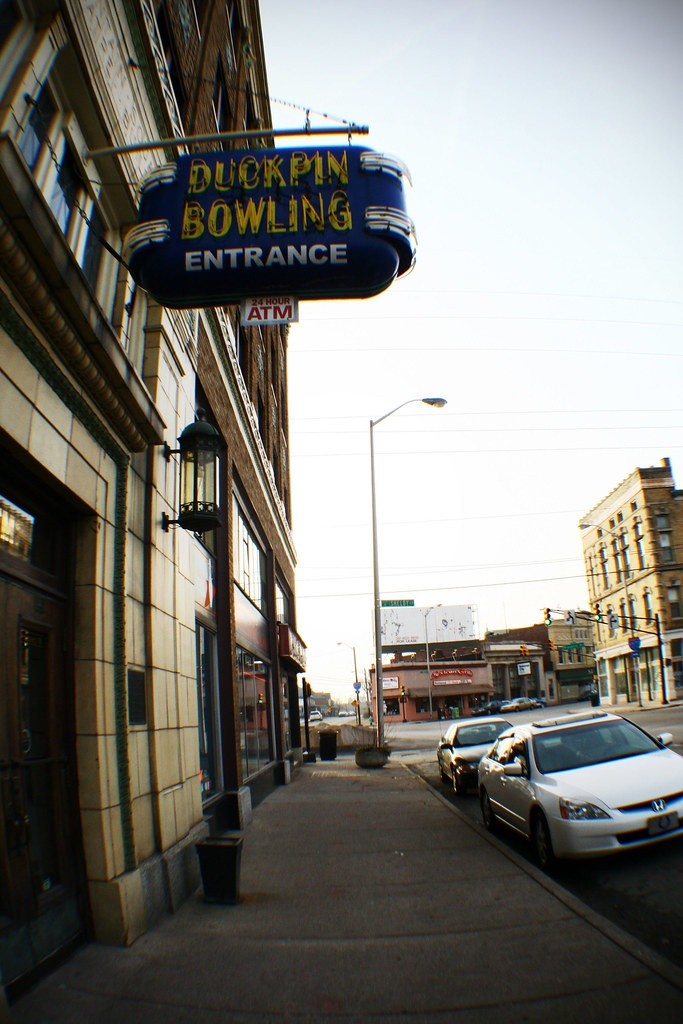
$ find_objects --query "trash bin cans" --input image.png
[194,836,245,905]
[590,694,600,707]
[318,730,339,762]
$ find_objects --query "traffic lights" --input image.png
[400,685,405,695]
[519,644,528,657]
[258,693,263,704]
[544,608,551,627]
[593,603,600,621]
[549,639,554,650]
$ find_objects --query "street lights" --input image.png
[337,643,361,725]
[578,524,643,707]
[369,396,447,747]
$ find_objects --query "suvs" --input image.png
[310,711,323,721]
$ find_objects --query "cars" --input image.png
[338,710,356,717]
[501,697,535,712]
[478,710,683,867]
[437,718,515,793]
[472,700,511,716]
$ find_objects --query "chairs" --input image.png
[542,744,580,771]
[586,732,610,757]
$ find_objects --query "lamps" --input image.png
[161,407,226,537]
[430,648,479,663]
